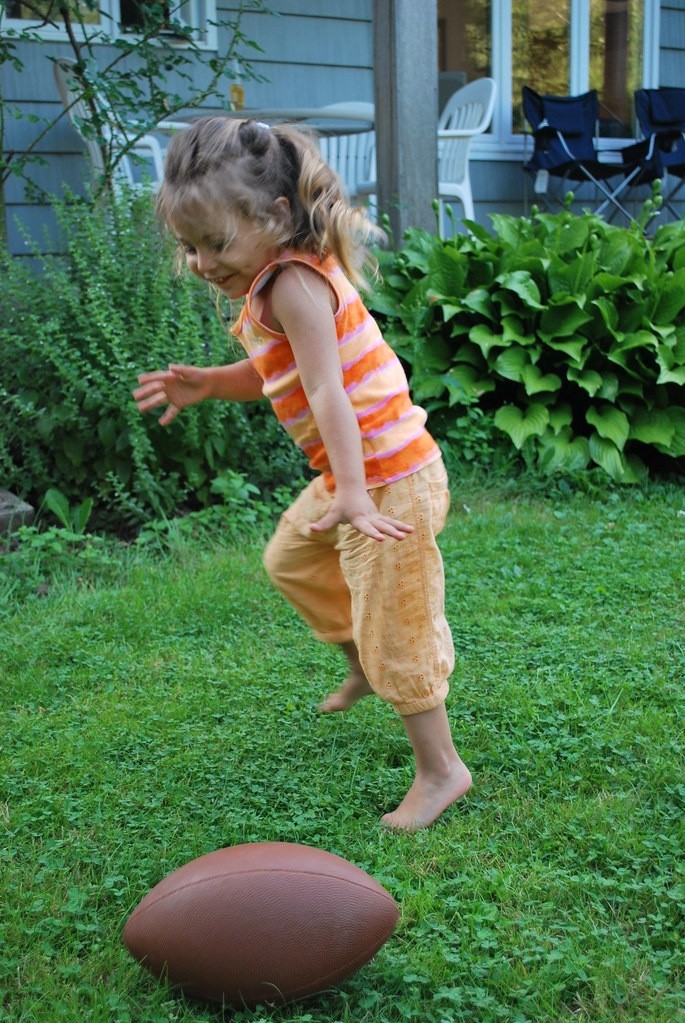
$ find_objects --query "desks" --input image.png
[120,111,374,140]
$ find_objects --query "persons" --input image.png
[132,118,473,832]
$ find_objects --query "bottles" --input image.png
[228,56,246,112]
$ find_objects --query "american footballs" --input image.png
[120,839,402,1010]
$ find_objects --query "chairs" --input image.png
[52,55,192,233]
[298,98,376,223]
[520,85,685,235]
[360,76,499,240]
